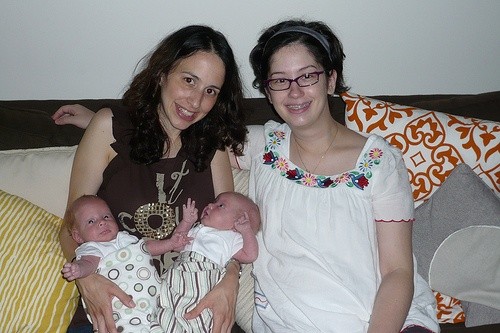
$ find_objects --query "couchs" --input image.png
[0,88,500,333]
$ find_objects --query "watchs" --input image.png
[225,258,242,279]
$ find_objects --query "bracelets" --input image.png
[71,257,76,263]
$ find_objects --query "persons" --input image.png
[52,21,439,333]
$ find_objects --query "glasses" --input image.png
[263,70,325,92]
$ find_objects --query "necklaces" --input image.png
[294,120,339,173]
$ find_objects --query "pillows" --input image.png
[340,92,500,324]
[0,145,79,219]
[411,163,500,327]
[0,190,82,333]
